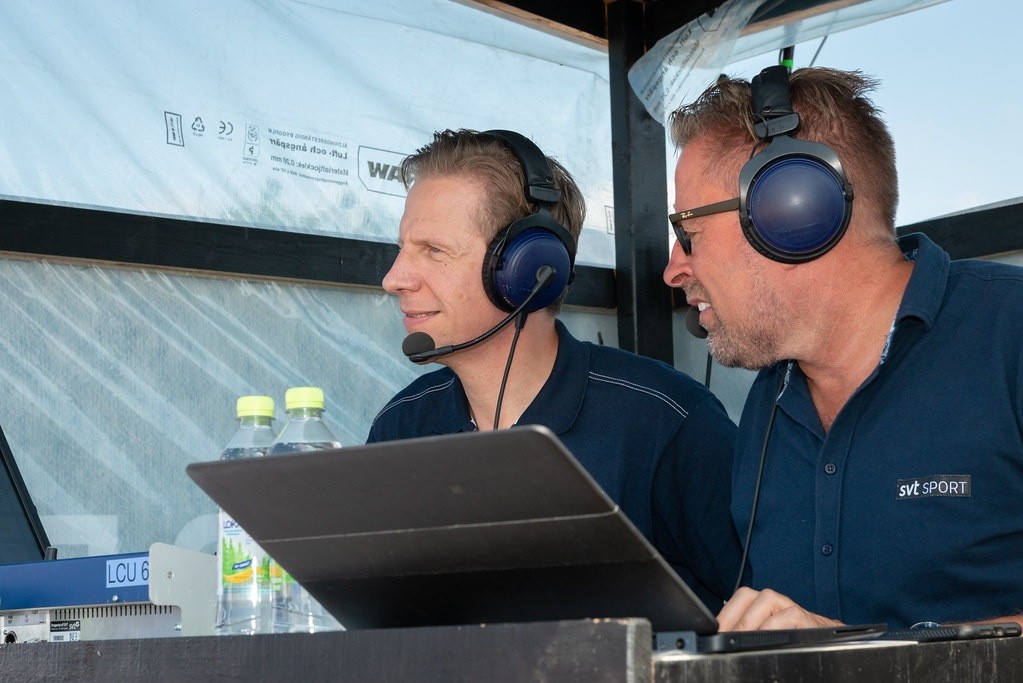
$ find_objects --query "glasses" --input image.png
[669,197,742,257]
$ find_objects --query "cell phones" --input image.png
[877,619,1021,642]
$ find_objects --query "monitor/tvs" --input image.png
[0,424,56,569]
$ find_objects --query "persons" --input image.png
[662,65,1022,632]
[362,129,742,615]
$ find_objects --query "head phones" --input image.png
[736,63,853,268]
[479,129,578,319]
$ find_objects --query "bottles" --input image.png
[217,396,277,637]
[267,388,344,631]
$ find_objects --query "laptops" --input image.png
[184,422,890,656]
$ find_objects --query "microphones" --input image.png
[685,305,708,340]
[401,265,555,365]
